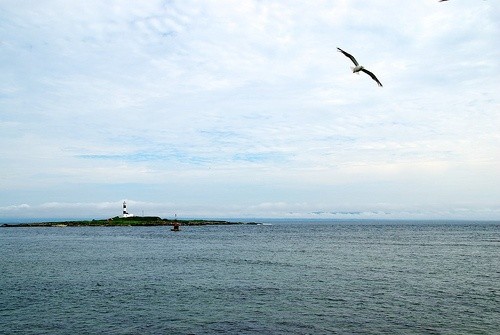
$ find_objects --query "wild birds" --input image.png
[337,47,383,86]
